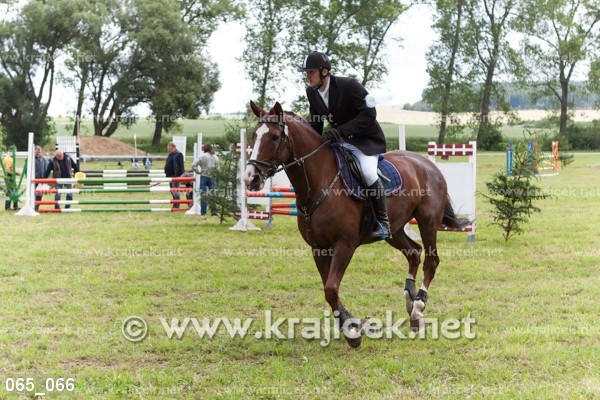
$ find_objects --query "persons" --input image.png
[3,145,22,211]
[24,145,48,211]
[44,149,81,208]
[142,151,152,170]
[190,143,221,216]
[164,141,185,208]
[297,51,393,240]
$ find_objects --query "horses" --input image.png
[244,99,476,349]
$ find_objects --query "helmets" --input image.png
[303,51,331,72]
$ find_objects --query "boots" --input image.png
[367,178,393,239]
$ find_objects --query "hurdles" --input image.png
[70,135,185,193]
[234,142,272,222]
[16,132,204,217]
[427,141,476,243]
[231,128,414,231]
